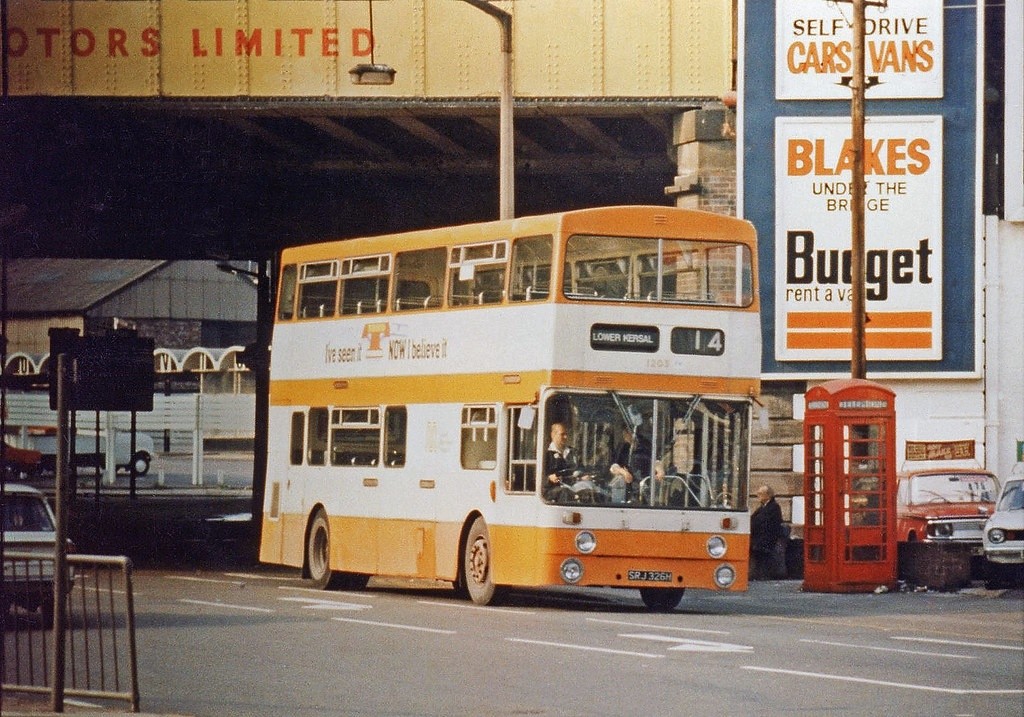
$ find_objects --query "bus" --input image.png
[258,206,760,610]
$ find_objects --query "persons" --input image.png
[543,423,603,503]
[617,425,657,490]
[749,485,782,580]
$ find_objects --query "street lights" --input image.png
[214,262,260,280]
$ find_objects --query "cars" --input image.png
[1,483,79,617]
[4,424,155,476]
[980,462,1024,590]
[894,467,1002,592]
